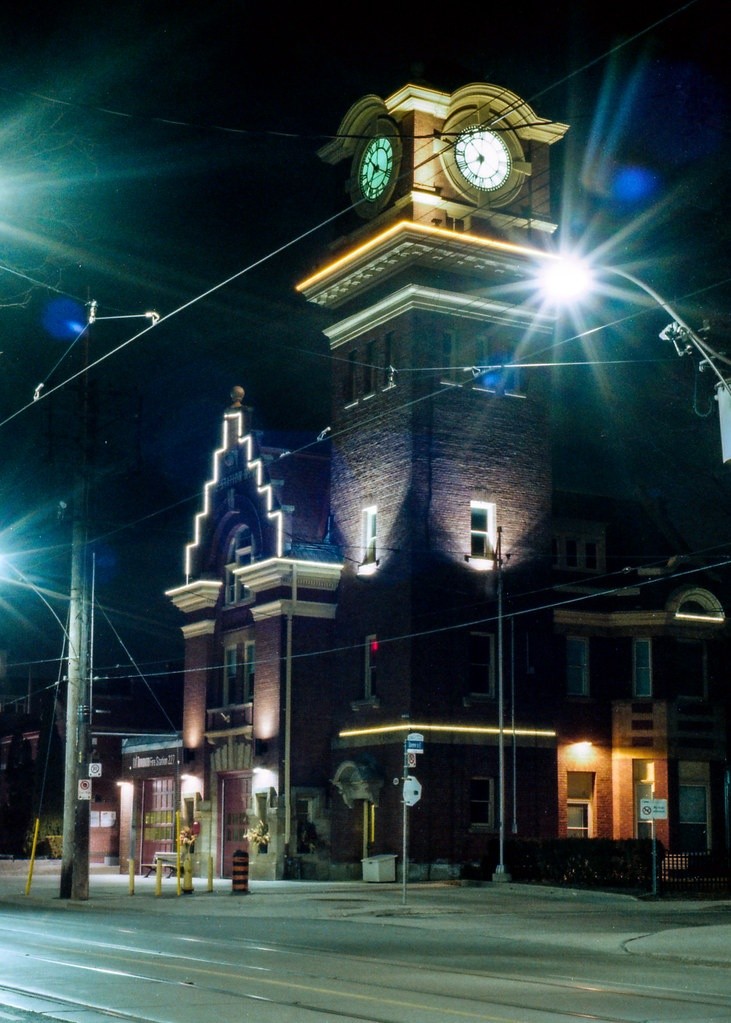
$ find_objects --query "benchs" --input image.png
[140,852,184,879]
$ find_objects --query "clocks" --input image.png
[455,123,511,192]
[357,133,394,202]
